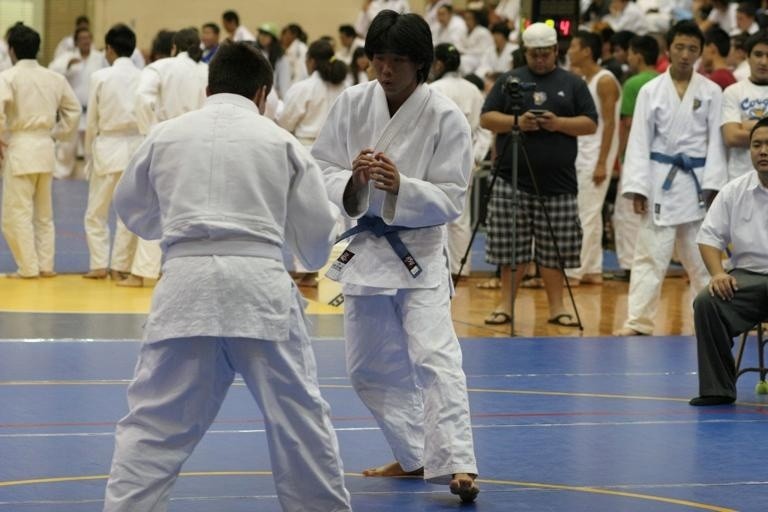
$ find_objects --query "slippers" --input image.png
[548,314,578,326]
[485,312,511,324]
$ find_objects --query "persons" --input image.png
[690,115,768,405]
[313,8,480,503]
[1,0,767,335]
[103,39,350,512]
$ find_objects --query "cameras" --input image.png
[504,75,536,108]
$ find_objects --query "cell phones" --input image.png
[531,109,544,115]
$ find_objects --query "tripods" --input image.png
[451,111,585,337]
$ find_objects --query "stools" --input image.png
[734,322,768,395]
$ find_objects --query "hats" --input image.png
[523,22,558,48]
[257,22,279,40]
